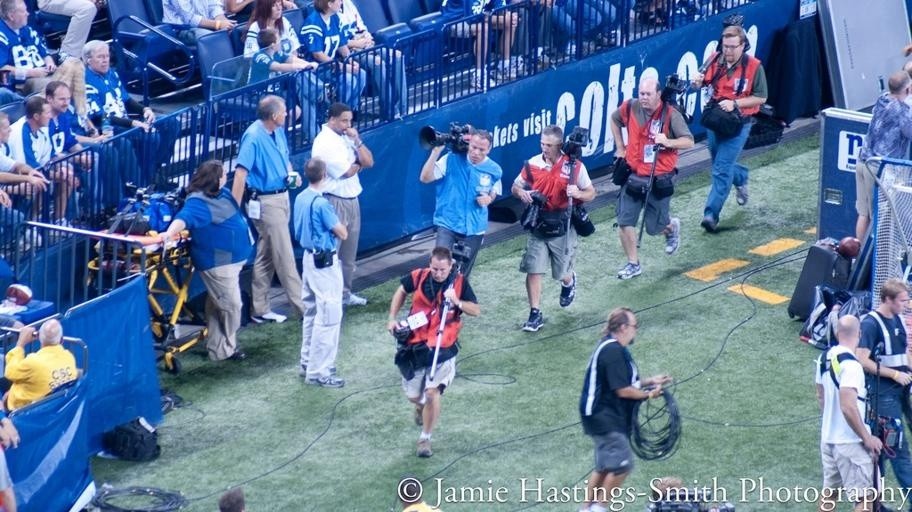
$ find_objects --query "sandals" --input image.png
[639,7,667,26]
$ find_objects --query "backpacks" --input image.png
[107,181,187,238]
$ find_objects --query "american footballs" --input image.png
[7,284,33,305]
[839,237,860,256]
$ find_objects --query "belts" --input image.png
[249,189,288,196]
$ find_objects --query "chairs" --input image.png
[1,1,748,325]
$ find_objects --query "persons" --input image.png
[0,0,911,512]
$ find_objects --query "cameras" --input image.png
[524,193,547,233]
[452,240,472,263]
[665,74,688,94]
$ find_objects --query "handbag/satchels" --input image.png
[535,209,570,237]
[799,284,872,349]
[610,158,674,200]
[700,96,743,138]
[391,320,412,345]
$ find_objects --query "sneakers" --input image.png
[342,294,372,306]
[736,184,748,206]
[559,271,576,308]
[228,352,247,360]
[616,260,642,280]
[250,312,288,322]
[664,217,680,254]
[522,307,544,332]
[700,214,716,231]
[414,400,423,425]
[416,437,433,458]
[299,364,344,388]
[472,32,616,90]
